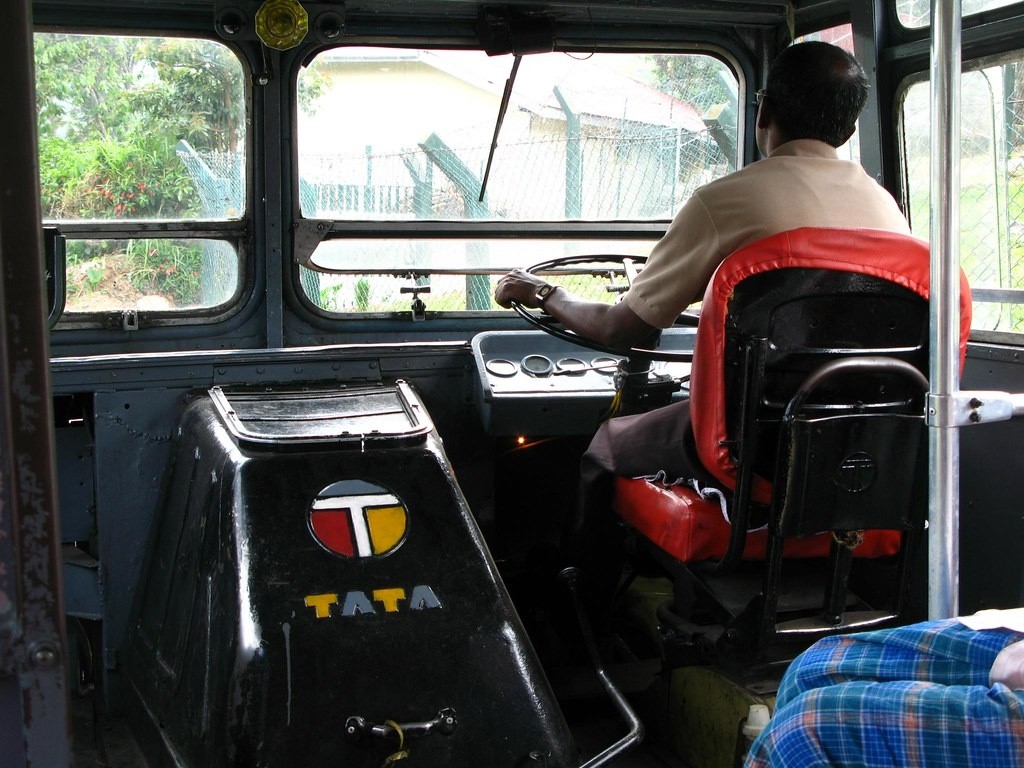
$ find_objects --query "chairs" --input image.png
[616,228,972,697]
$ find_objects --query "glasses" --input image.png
[754,89,766,102]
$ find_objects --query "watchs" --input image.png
[535,282,562,312]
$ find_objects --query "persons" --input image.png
[743,606,1024,768]
[493,42,911,653]
